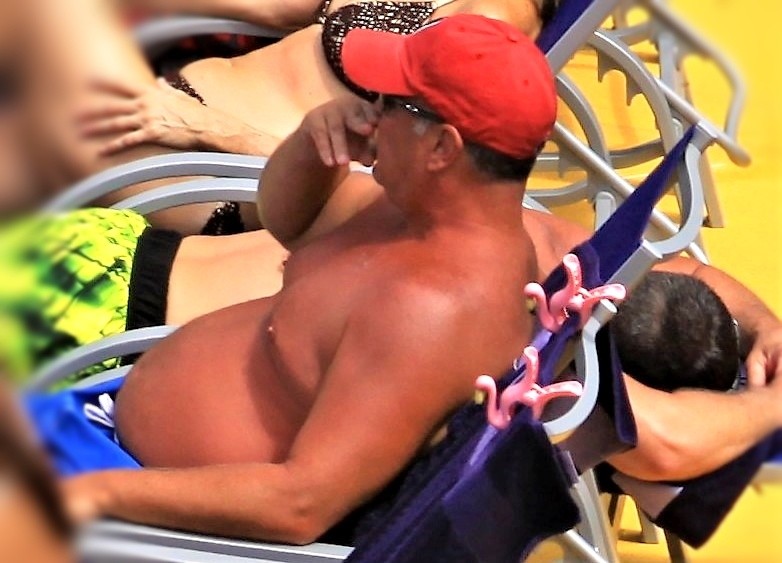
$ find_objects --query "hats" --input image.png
[342,14,557,159]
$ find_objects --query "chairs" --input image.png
[19,0,752,563]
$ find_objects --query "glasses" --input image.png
[383,94,447,124]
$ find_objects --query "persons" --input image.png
[0,0,564,563]
[587,256,781,482]
